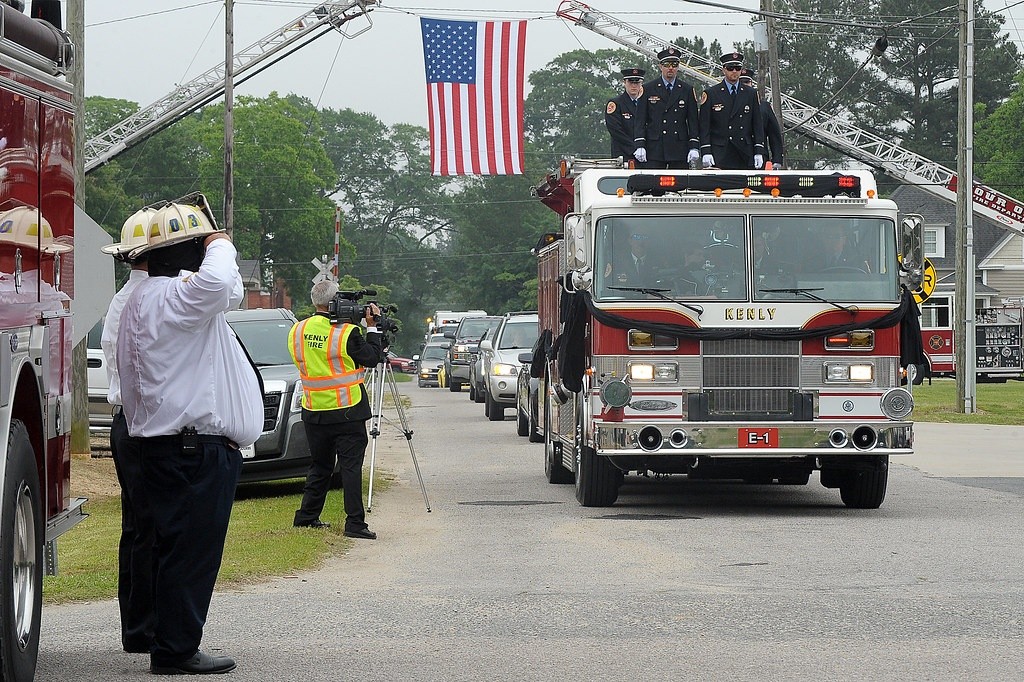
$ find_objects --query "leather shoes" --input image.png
[295,519,330,527]
[151,651,235,674]
[343,527,377,540]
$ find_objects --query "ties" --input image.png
[632,99,636,106]
[225,319,267,399]
[666,83,672,93]
[731,86,738,104]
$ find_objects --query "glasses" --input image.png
[662,61,679,68]
[725,66,741,71]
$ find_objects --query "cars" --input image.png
[413,342,452,388]
[441,331,457,388]
[470,327,499,403]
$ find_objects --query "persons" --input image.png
[799,220,873,274]
[665,241,727,301]
[1,204,72,520]
[701,52,766,172]
[102,204,162,654]
[740,69,786,170]
[731,231,788,301]
[635,50,701,172]
[605,221,682,303]
[606,70,646,163]
[115,203,266,677]
[287,282,379,540]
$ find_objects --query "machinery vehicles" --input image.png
[537,156,926,508]
[0,0,76,681]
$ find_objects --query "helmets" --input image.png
[129,203,227,260]
[101,208,158,255]
[0,206,73,255]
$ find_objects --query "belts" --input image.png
[145,433,239,451]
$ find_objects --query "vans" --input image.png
[425,333,454,342]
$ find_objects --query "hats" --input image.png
[738,69,754,80]
[658,49,682,61]
[620,69,645,80]
[720,53,742,67]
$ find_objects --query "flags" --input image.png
[419,14,529,177]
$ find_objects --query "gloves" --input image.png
[702,153,715,167]
[634,148,647,162]
[754,154,763,169]
[772,163,782,169]
[687,150,699,163]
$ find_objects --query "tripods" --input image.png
[338,353,432,514]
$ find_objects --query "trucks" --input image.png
[423,311,487,334]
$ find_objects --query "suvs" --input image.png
[480,310,538,421]
[224,309,342,488]
[364,351,417,374]
[444,316,505,392]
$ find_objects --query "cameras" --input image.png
[329,289,399,352]
